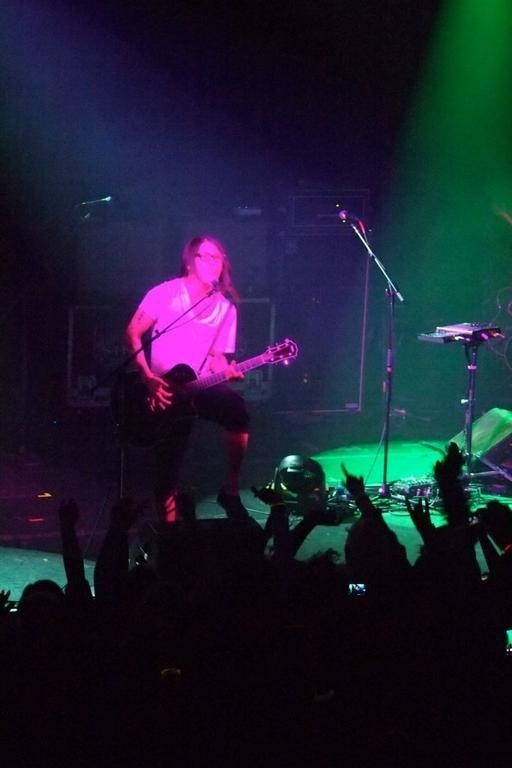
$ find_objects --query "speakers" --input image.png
[448,406,512,473]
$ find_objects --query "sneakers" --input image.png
[217,487,249,519]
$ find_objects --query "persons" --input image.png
[122,232,252,528]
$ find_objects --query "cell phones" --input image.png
[347,581,366,597]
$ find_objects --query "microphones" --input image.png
[79,196,112,208]
[330,193,349,224]
[209,275,237,308]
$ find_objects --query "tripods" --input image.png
[340,225,441,517]
[443,353,512,489]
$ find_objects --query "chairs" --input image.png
[79,371,194,558]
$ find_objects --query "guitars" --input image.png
[108,337,299,451]
